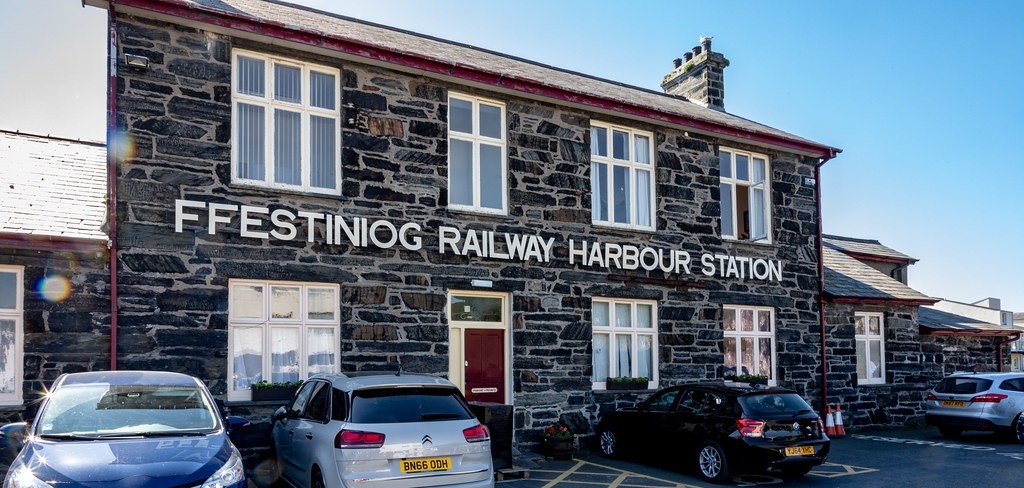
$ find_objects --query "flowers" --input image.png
[543,421,574,439]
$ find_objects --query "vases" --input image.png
[543,435,575,461]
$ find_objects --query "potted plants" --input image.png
[251,380,305,402]
[732,374,768,386]
[606,376,649,390]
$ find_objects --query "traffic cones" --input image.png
[825,405,836,436]
[835,403,847,436]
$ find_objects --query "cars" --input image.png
[267,370,496,488]
[0,370,252,488]
[927,370,1024,445]
[594,382,832,483]
